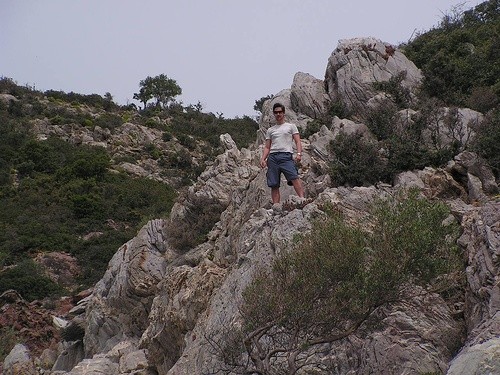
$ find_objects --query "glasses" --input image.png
[273,111,283,114]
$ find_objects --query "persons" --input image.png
[260,103,304,205]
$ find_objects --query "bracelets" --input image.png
[297,152,301,156]
